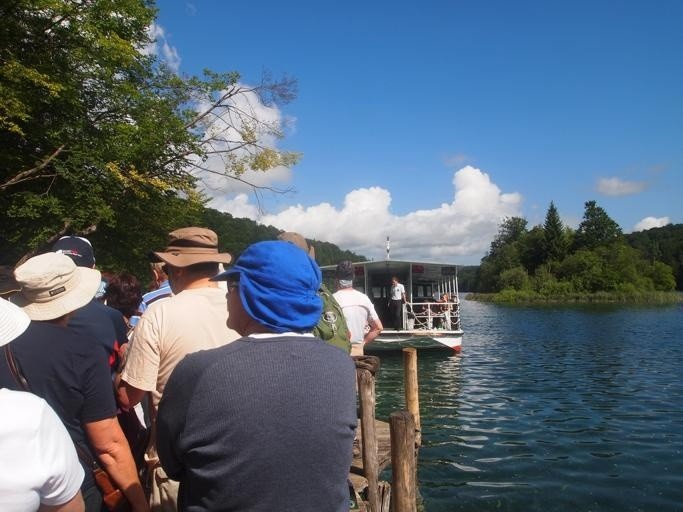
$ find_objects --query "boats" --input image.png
[315,236,463,353]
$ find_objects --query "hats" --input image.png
[155,227,232,267]
[336,261,355,280]
[7,251,102,321]
[150,247,165,262]
[277,232,315,260]
[0,296,30,346]
[208,240,323,333]
[52,236,95,267]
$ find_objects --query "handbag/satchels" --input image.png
[92,466,126,512]
